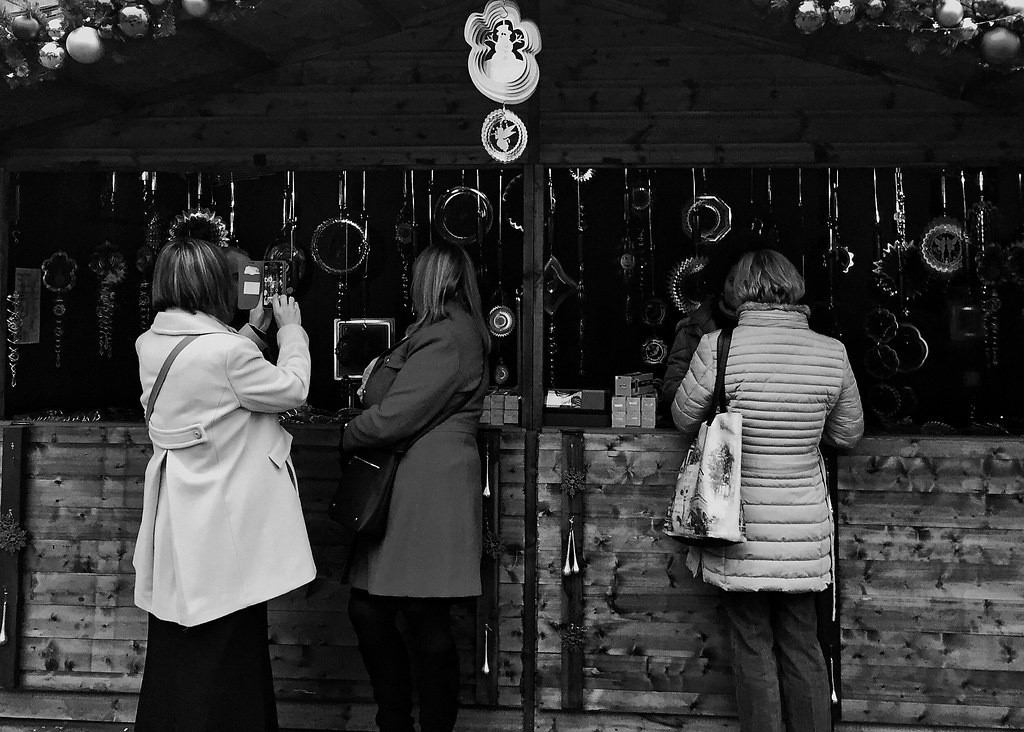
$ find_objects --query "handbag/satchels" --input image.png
[327,447,406,541]
[662,327,744,547]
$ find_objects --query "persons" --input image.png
[662,249,866,732]
[131,236,317,732]
[339,242,491,732]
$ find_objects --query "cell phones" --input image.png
[262,260,286,310]
